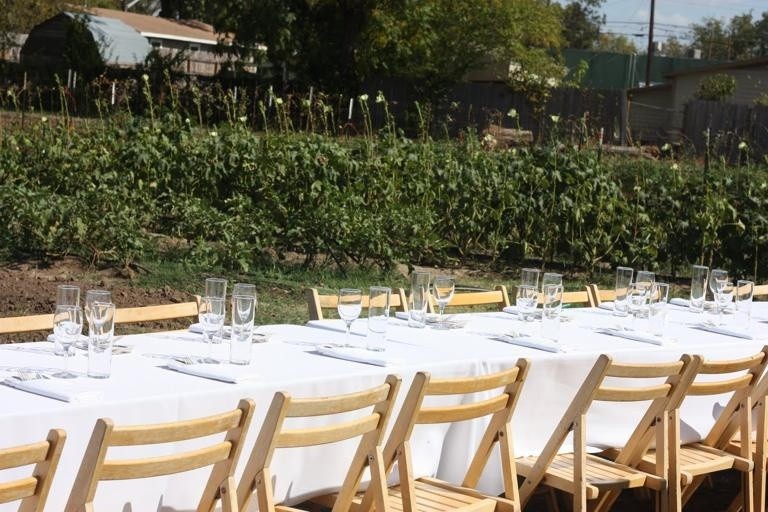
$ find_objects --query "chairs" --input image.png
[732,282,768,303]
[418,286,512,313]
[514,353,694,512]
[514,286,594,309]
[235,374,402,512]
[361,357,531,512]
[591,283,662,308]
[65,397,255,512]
[0,429,66,512]
[601,340,768,512]
[308,288,410,320]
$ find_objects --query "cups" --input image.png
[202,276,228,345]
[407,271,431,329]
[732,280,755,329]
[86,300,116,379]
[540,284,564,339]
[688,264,709,312]
[517,268,540,323]
[54,284,81,358]
[612,266,633,317]
[228,293,256,365]
[647,283,670,337]
[364,285,392,352]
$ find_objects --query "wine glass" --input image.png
[430,274,457,331]
[230,283,257,355]
[541,272,563,325]
[709,281,735,317]
[635,271,655,319]
[83,289,111,358]
[51,303,84,380]
[336,288,363,350]
[706,268,729,315]
[624,282,647,330]
[515,285,539,338]
[196,295,226,366]
